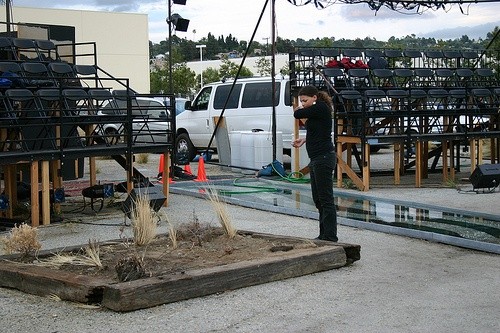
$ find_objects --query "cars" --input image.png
[342,104,496,155]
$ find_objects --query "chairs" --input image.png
[299,50,500,136]
[0,37,155,152]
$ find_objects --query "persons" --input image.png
[291,85,339,243]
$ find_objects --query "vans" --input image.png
[172,77,336,163]
[97,95,193,145]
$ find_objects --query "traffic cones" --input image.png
[158,154,174,184]
[193,158,208,181]
[184,164,193,175]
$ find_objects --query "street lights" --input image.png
[196,45,207,89]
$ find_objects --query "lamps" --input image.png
[166,13,190,32]
[172,0,186,5]
[81,182,114,212]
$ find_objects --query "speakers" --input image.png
[120,186,167,220]
[469,164,500,188]
